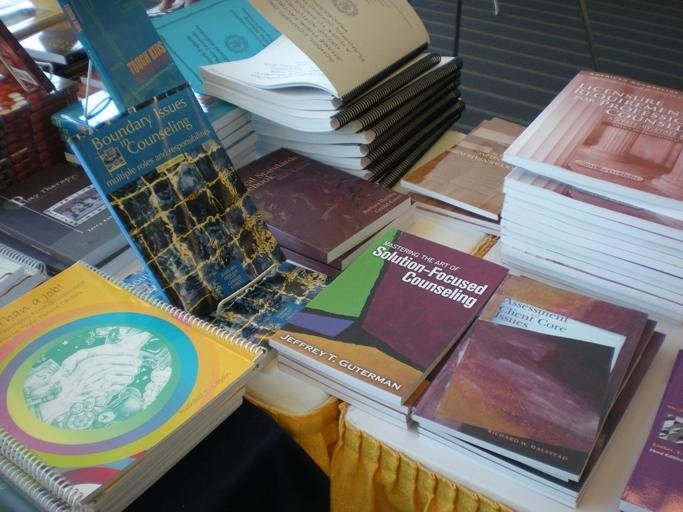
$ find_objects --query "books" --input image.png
[1,0,682,512]
